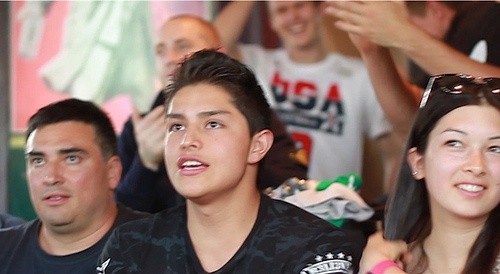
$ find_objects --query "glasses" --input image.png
[418,72,500,107]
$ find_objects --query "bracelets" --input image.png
[365,256,401,274]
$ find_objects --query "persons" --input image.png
[114,1,500,216]
[359,73,499,274]
[0,96,151,274]
[94,46,361,274]
